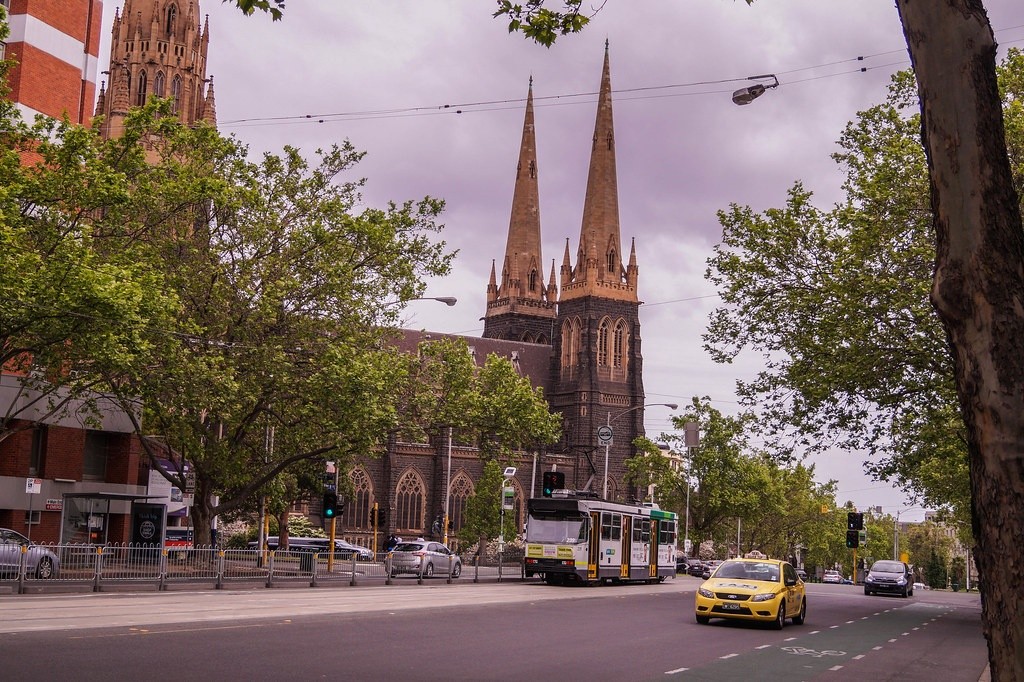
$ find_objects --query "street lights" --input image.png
[603,403,678,500]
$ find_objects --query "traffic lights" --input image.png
[448,521,453,529]
[846,531,858,548]
[323,493,335,518]
[543,472,565,498]
[379,509,385,526]
[848,512,856,529]
[336,502,344,516]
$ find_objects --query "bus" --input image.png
[525,498,679,587]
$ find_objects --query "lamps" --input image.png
[732,82,779,104]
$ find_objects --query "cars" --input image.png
[0,528,59,579]
[247,536,374,562]
[695,558,806,629]
[843,578,854,584]
[864,560,913,598]
[822,571,843,584]
[386,541,462,578]
[687,560,722,580]
[795,570,806,582]
